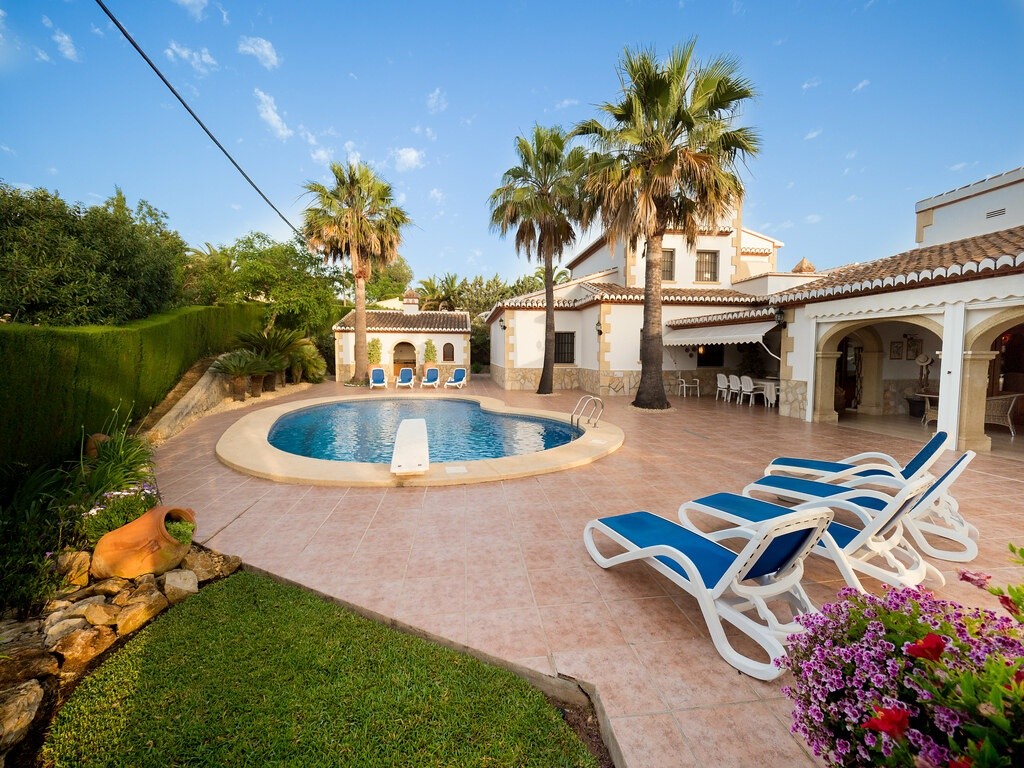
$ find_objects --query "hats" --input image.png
[916,354,932,366]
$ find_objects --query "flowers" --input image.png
[773,542,1024,768]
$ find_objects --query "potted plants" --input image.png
[423,339,438,376]
[367,338,382,378]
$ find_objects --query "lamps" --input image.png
[498,317,507,330]
[775,309,787,329]
[596,321,603,335]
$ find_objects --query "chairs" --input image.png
[984,393,1024,436]
[716,373,730,401]
[584,508,833,682]
[369,368,387,389]
[728,375,753,404]
[420,368,440,389]
[677,371,700,398]
[444,368,467,389]
[396,367,414,388]
[764,431,959,512]
[772,386,780,407]
[677,474,944,605]
[741,450,980,562]
[740,376,767,407]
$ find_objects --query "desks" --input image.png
[915,392,939,427]
[721,378,780,407]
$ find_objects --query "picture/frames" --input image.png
[890,341,903,360]
[906,339,923,360]
